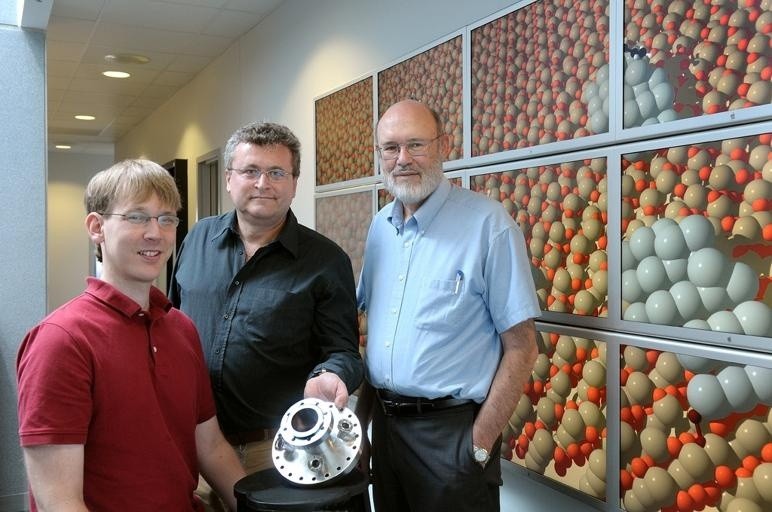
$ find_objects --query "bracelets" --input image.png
[308,368,331,380]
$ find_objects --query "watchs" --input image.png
[473,445,490,464]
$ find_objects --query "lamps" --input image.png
[99,54,136,81]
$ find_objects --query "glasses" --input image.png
[101,210,182,231]
[228,165,297,184]
[376,132,447,158]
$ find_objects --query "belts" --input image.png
[221,426,278,447]
[377,394,472,417]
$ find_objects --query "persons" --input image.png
[169,120,364,512]
[358,100,539,512]
[17,158,247,511]
